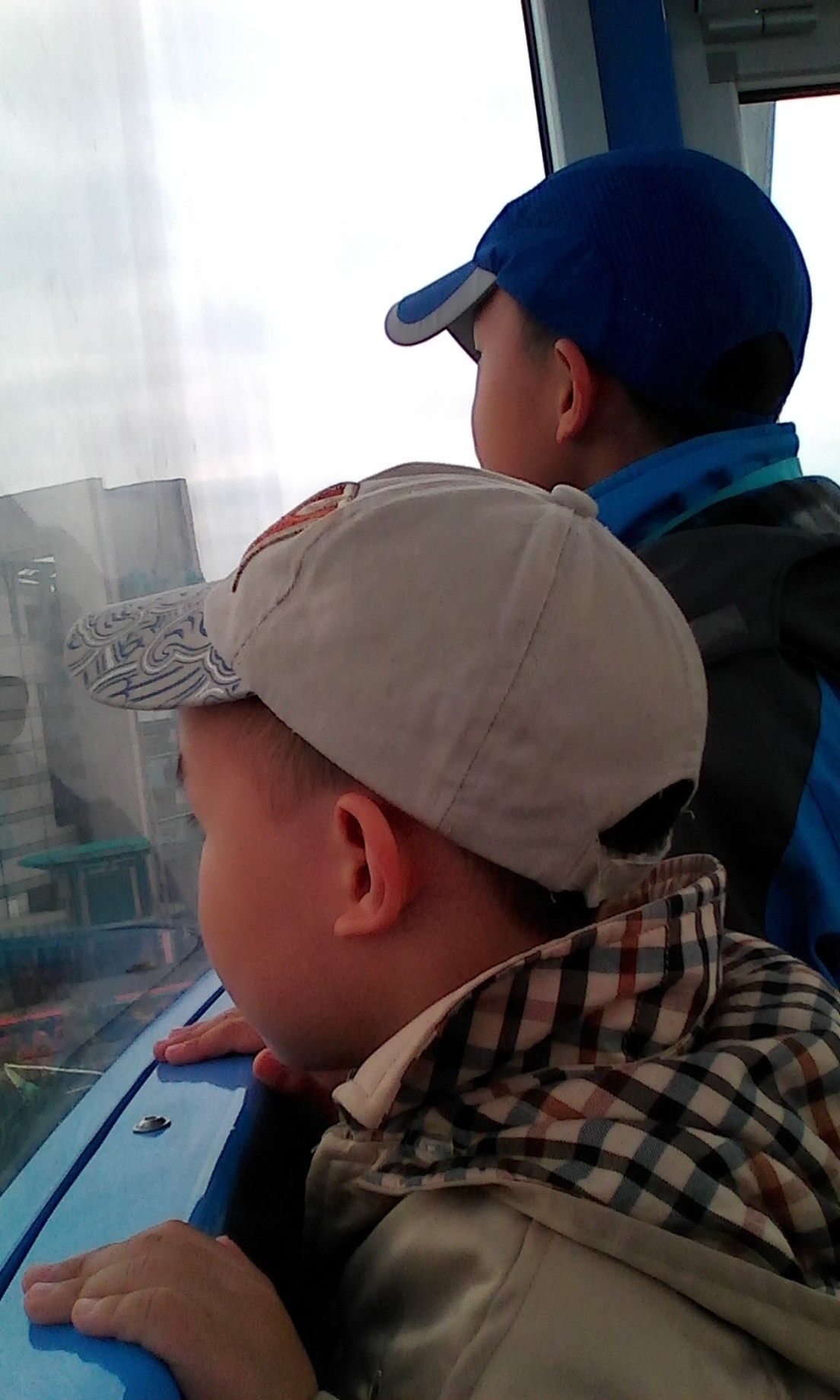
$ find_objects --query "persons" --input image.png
[385,147,840,992]
[19,458,840,1399]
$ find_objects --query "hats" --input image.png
[386,146,811,450]
[63,461,706,905]
